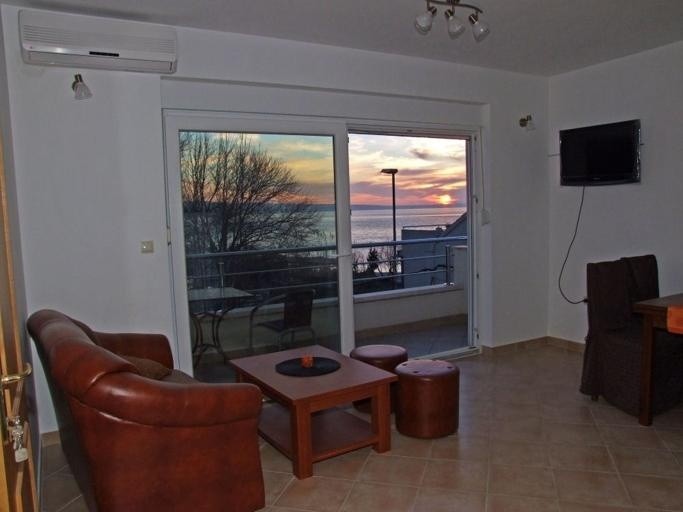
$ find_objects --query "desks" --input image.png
[633,293,683,426]
[188,286,257,369]
[229,344,399,479]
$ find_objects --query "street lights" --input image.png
[381,168,399,274]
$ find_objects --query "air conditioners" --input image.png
[18,10,178,74]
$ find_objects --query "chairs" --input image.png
[621,255,683,410]
[580,260,675,416]
[250,289,317,353]
[27,309,263,512]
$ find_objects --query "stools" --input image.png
[394,359,459,440]
[349,345,407,414]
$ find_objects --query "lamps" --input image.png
[520,115,535,131]
[415,0,490,43]
[73,74,92,100]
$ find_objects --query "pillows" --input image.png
[120,356,171,379]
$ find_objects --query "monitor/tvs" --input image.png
[558,118,641,187]
